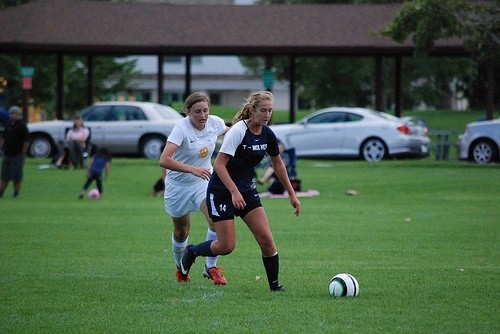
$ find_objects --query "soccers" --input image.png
[328,272,359,298]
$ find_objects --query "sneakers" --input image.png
[179,244,196,278]
[203,264,227,285]
[175,263,191,283]
[270,285,286,292]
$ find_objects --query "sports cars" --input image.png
[263,106,431,164]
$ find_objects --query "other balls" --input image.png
[88,188,100,199]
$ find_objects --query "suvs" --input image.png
[23,101,186,160]
[456,116,500,166]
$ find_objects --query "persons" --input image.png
[0,106,29,198]
[51,115,90,171]
[257,140,301,197]
[78,147,111,199]
[159,92,230,286]
[180,90,301,291]
